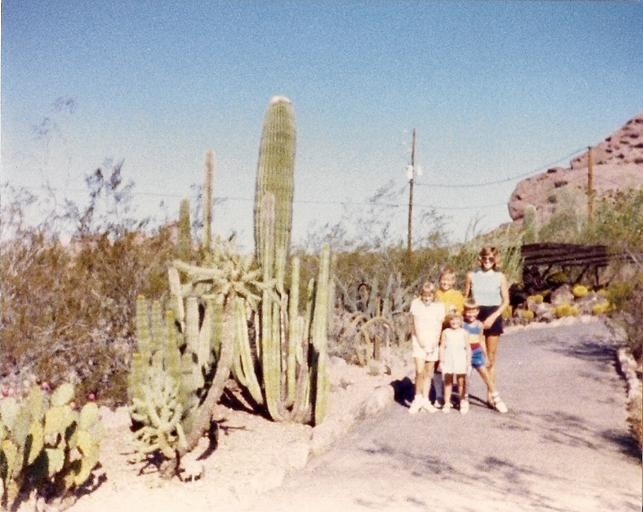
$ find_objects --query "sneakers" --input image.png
[408,392,509,415]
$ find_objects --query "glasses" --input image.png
[482,256,495,263]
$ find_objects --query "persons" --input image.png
[409,282,445,412]
[433,269,464,408]
[460,300,507,414]
[463,246,509,407]
[440,308,472,414]
